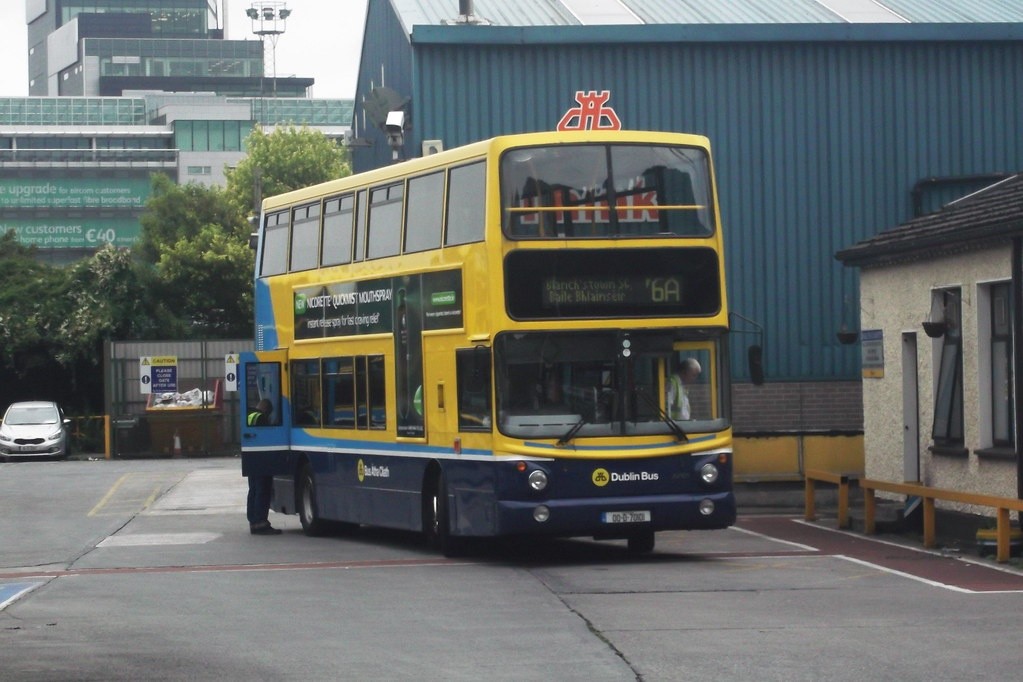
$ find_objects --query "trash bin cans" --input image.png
[113,414,151,458]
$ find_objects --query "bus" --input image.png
[237,128,770,561]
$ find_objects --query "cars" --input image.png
[0,400,72,460]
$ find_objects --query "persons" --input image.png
[241,398,283,534]
[665,358,701,421]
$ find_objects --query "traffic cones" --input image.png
[171,426,183,459]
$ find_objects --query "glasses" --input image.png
[543,367,554,374]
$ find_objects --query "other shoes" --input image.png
[250,523,255,533]
[254,520,282,535]
